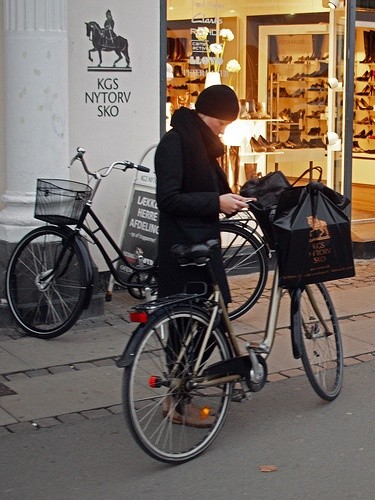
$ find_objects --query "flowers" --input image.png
[196,27,241,86]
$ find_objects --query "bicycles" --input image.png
[4,148,273,340]
[116,171,345,464]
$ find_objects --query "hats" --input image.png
[195,84,240,123]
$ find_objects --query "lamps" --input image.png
[328,0,340,9]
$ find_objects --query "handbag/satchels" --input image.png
[241,171,293,251]
[272,166,356,290]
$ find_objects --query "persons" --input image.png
[154,85,257,427]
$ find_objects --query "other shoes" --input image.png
[163,401,217,428]
[167,30,375,195]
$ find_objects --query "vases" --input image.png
[205,73,221,88]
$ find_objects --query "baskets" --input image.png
[34,178,92,224]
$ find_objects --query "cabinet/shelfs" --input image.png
[159,11,375,189]
